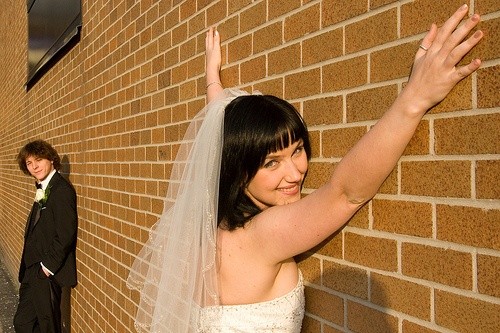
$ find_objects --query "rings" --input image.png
[418,43,428,51]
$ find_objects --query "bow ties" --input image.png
[34,181,41,189]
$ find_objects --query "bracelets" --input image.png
[206,82,224,88]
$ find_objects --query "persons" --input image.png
[12,139,77,333]
[127,3,483,333]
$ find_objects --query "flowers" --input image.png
[34,185,53,207]
